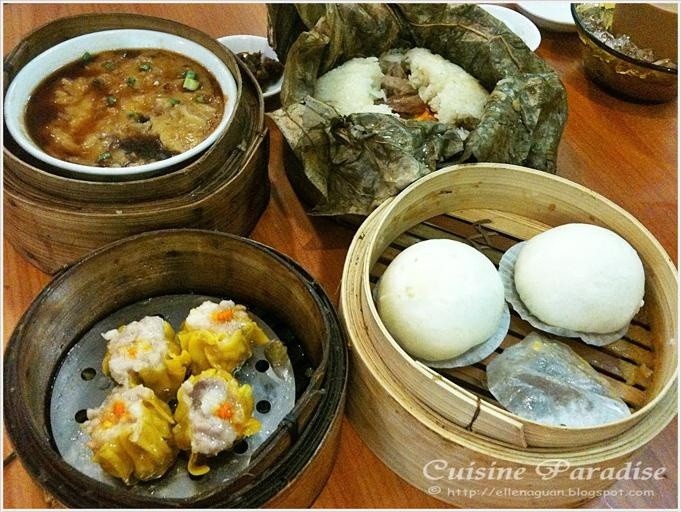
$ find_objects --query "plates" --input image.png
[216,35,286,98]
[4,29,238,180]
[477,3,576,52]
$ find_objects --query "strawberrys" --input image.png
[379,238,505,362]
[513,222,647,334]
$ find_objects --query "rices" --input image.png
[312,47,490,124]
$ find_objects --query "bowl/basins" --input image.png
[571,3,678,104]
